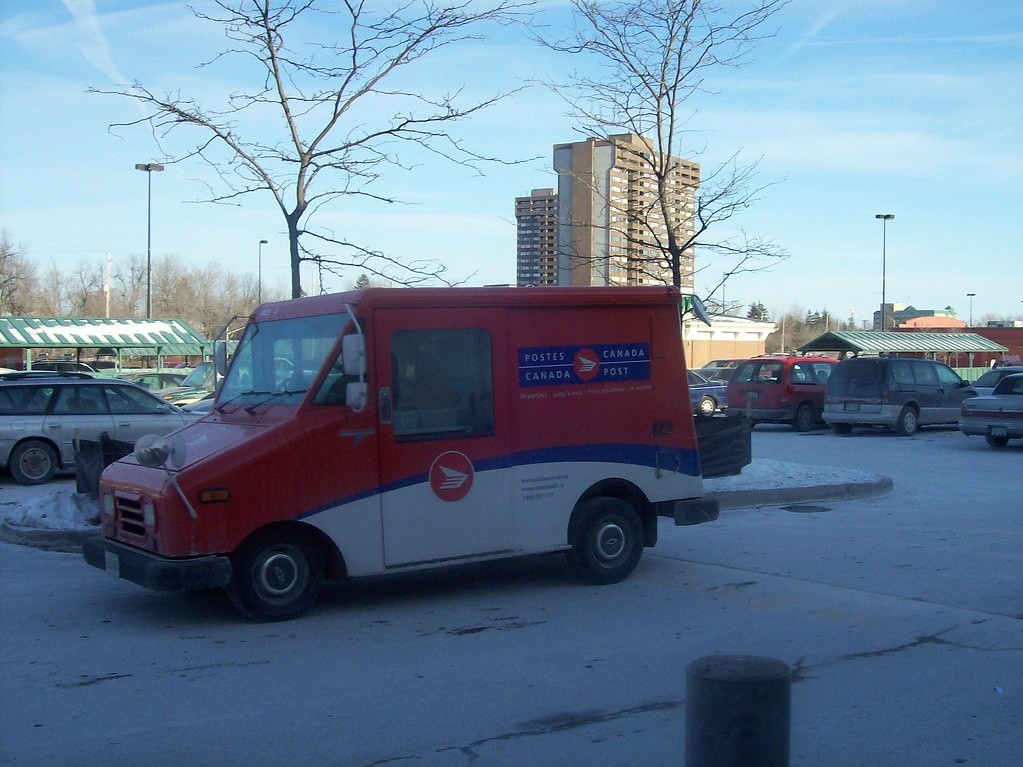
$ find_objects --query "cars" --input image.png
[687,353,812,418]
[958,373,1023,447]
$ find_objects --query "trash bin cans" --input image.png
[679,653,794,767]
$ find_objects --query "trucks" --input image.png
[82,286,720,623]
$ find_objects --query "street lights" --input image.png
[259,239,268,304]
[875,214,895,331]
[135,164,164,367]
[967,293,975,327]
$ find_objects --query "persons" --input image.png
[328,322,400,409]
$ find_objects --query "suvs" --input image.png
[0,353,342,486]
[821,354,970,436]
[722,350,841,432]
[971,360,1023,397]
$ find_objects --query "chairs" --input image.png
[67,395,83,410]
[81,398,101,413]
